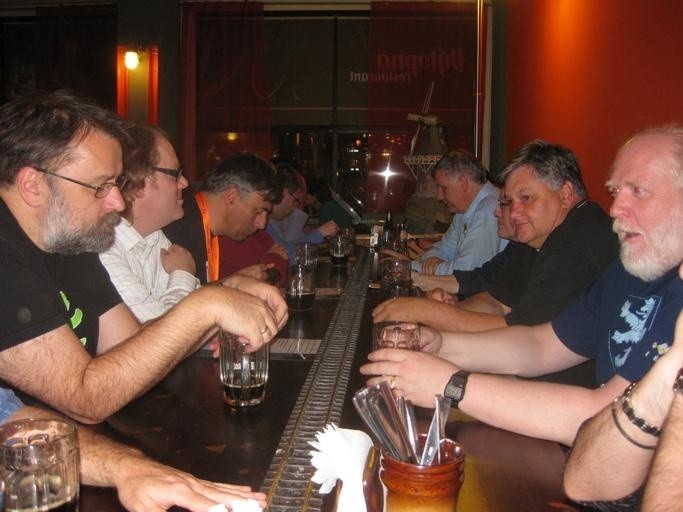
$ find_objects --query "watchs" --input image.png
[619,381,663,440]
[444,369,471,413]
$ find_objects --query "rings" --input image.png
[261,325,269,335]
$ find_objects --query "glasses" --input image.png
[497,199,511,209]
[32,164,129,199]
[287,192,305,210]
[152,165,182,182]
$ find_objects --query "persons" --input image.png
[0,384,270,512]
[265,161,346,268]
[370,138,622,389]
[0,89,290,425]
[378,147,511,277]
[160,151,284,287]
[421,178,516,303]
[559,259,682,512]
[359,124,682,449]
[94,122,206,326]
[218,154,307,288]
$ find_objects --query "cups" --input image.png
[297,242,318,272]
[381,217,410,257]
[377,432,466,507]
[285,255,318,310]
[327,228,354,269]
[1,416,80,512]
[369,222,383,248]
[382,260,410,297]
[372,322,421,353]
[218,329,269,408]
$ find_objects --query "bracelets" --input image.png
[611,400,659,451]
[217,278,230,288]
